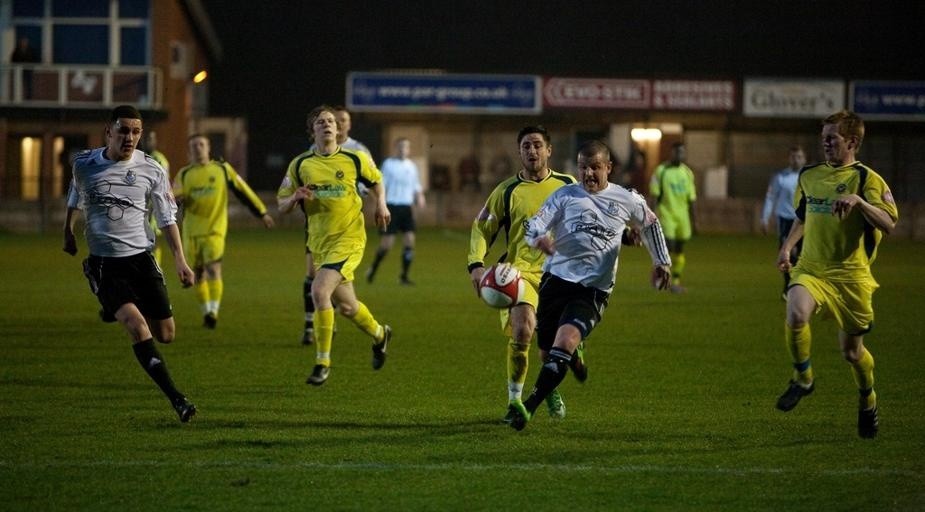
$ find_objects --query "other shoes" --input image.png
[204,310,217,329]
[401,274,410,284]
[367,270,376,283]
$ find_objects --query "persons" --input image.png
[301,108,375,347]
[467,127,569,421]
[277,106,392,385]
[140,127,172,270]
[367,136,425,288]
[777,111,899,440]
[645,142,698,294]
[170,136,275,328]
[503,140,673,430]
[760,147,811,301]
[63,105,198,422]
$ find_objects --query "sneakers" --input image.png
[306,364,331,385]
[507,343,587,431]
[302,321,314,344]
[858,406,878,438]
[172,395,196,422]
[775,380,815,411]
[332,329,337,340]
[372,324,392,370]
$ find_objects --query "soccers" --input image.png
[480,263,525,307]
